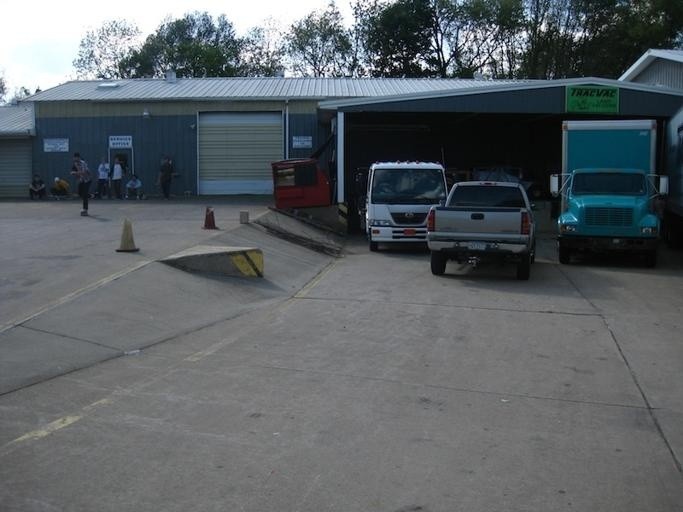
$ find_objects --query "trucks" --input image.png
[549,119,670,267]
[364,159,450,260]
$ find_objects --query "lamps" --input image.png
[143,101,150,118]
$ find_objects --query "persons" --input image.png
[157,156,171,200]
[109,157,123,200]
[123,175,143,200]
[49,176,68,199]
[96,157,111,199]
[28,174,45,199]
[70,152,91,214]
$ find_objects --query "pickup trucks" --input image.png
[426,181,537,281]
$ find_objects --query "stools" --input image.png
[184,190,191,200]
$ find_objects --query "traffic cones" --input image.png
[201,206,218,229]
[116,216,139,251]
